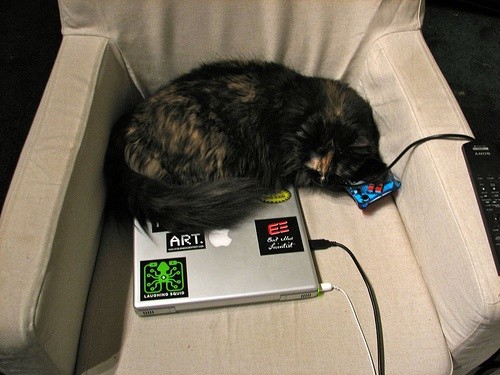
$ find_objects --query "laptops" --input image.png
[132,183,323,316]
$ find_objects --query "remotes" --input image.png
[463,139,500,276]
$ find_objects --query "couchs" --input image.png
[0,1,500,375]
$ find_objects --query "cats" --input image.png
[100,48,391,235]
